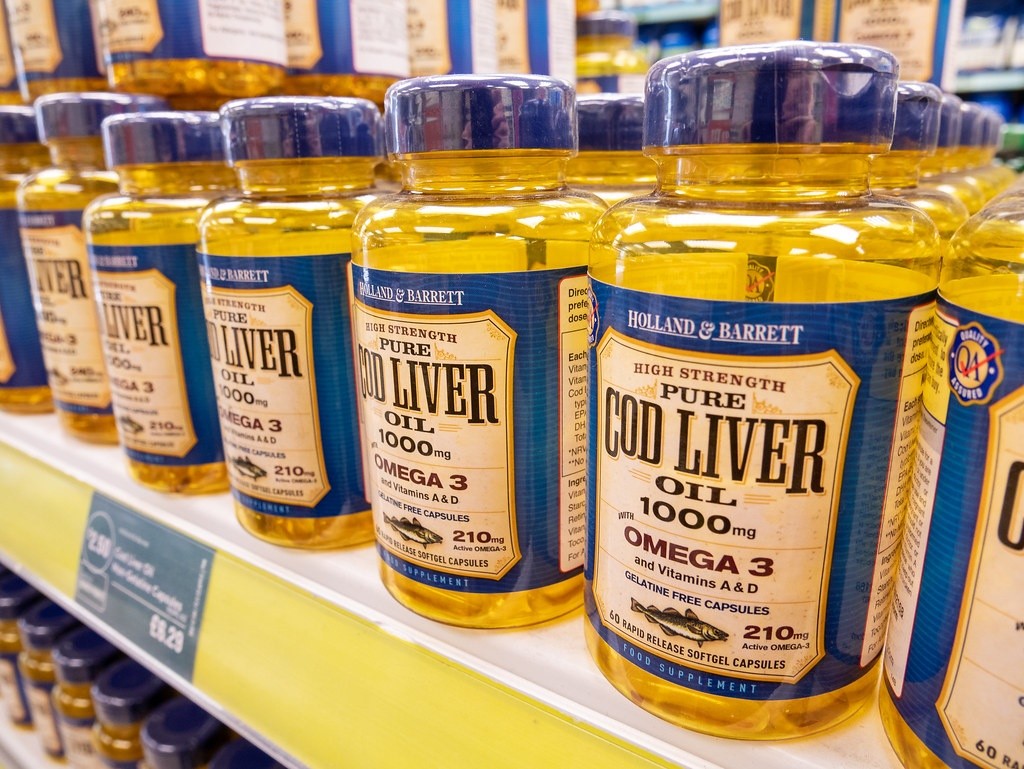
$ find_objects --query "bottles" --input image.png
[0,0,1024,769]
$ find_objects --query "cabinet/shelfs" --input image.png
[0,405,907,769]
